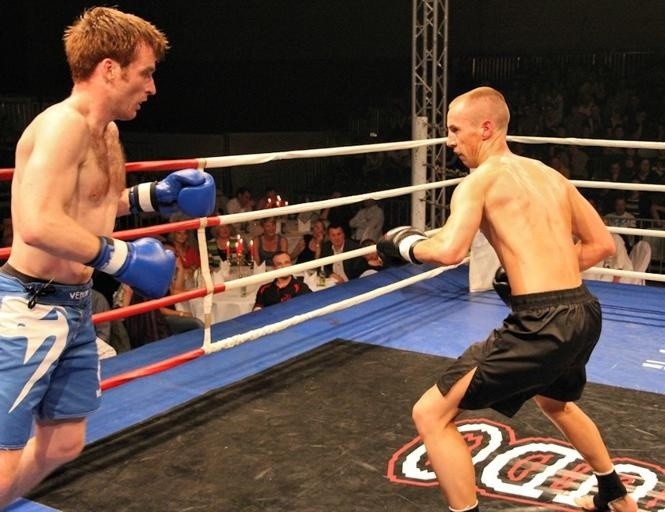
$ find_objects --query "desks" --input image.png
[173,266,335,327]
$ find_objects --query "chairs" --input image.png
[628,240,651,286]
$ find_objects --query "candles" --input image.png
[226,234,254,258]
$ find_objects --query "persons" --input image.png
[92,186,413,360]
[444,68,665,290]
[383,86,639,512]
[0,7,217,512]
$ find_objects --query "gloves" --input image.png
[492,267,511,304]
[84,236,176,298]
[376,225,430,268]
[129,168,216,217]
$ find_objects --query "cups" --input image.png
[320,271,325,286]
